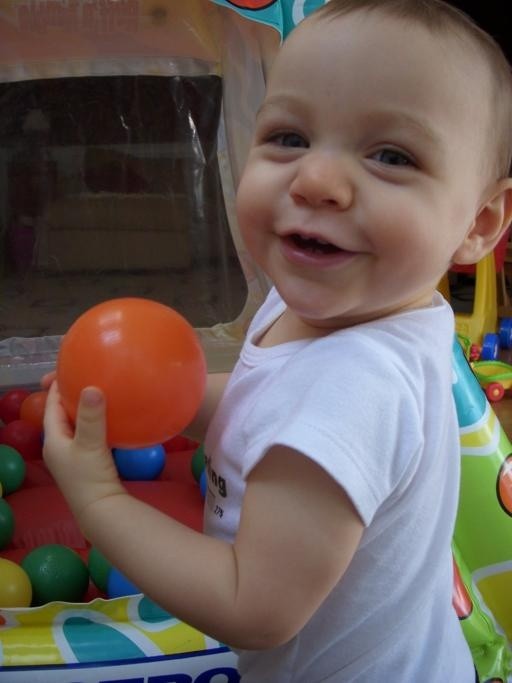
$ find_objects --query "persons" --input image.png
[40,0,512,683]
